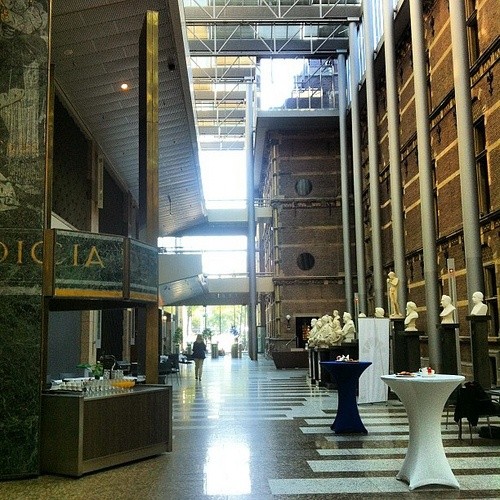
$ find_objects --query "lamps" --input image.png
[286,314,291,328]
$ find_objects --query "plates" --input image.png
[396,375,416,377]
[336,360,344,362]
[422,375,436,377]
[348,361,358,362]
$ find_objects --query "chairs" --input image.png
[137,353,182,385]
[445,381,500,445]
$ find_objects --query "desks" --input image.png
[380,374,465,491]
[39,383,173,480]
[318,361,373,435]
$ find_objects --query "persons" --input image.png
[470,291,488,316]
[387,272,403,317]
[358,313,366,318]
[404,301,419,331]
[192,334,206,381]
[439,295,457,324]
[306,310,356,348]
[374,307,385,317]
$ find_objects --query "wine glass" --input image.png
[111,379,135,392]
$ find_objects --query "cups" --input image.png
[85,380,118,392]
[419,367,427,375]
